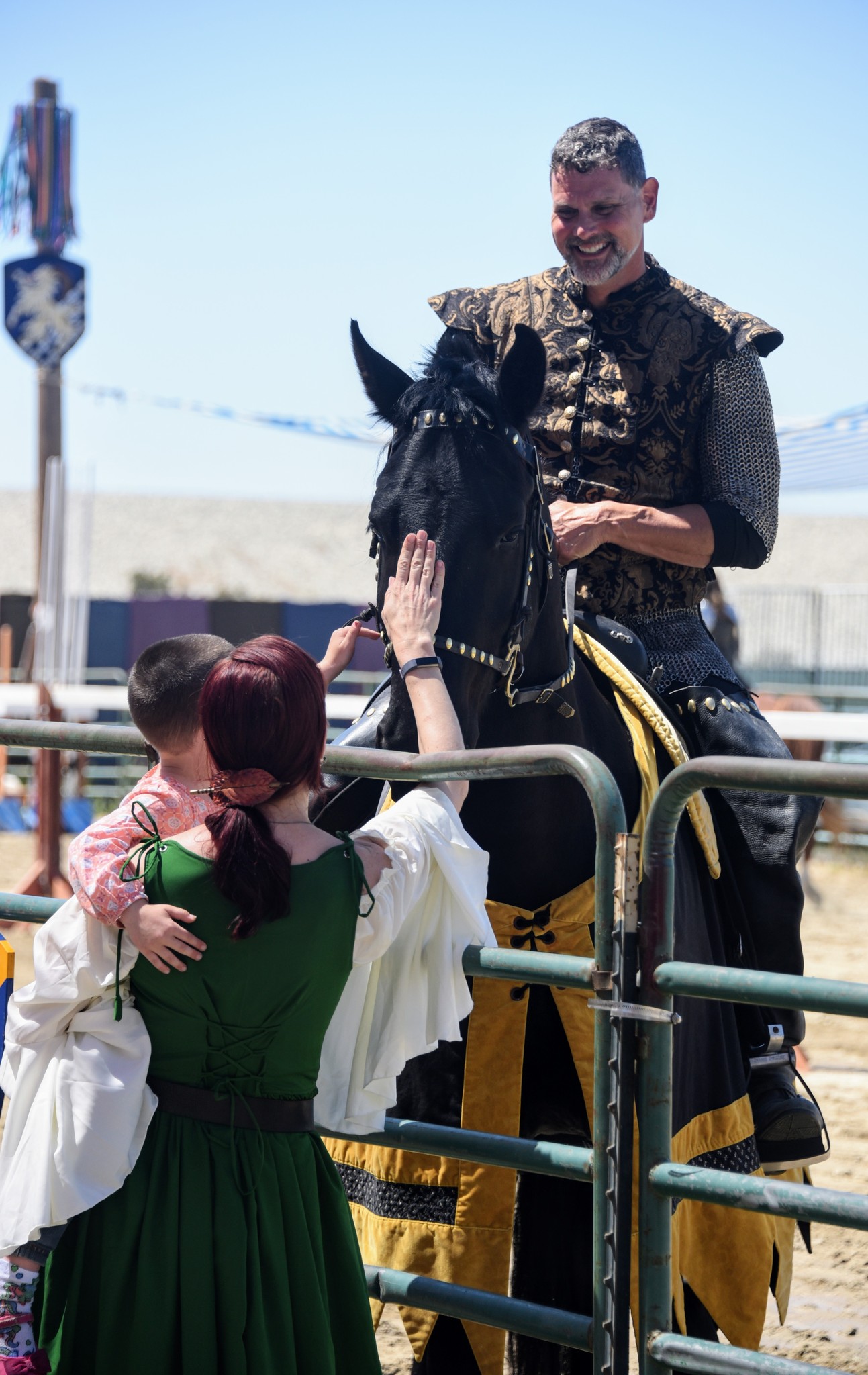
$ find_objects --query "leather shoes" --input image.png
[751,1063,825,1152]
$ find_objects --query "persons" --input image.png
[307,119,827,1173]
[0,528,506,1375]
[0,622,381,1375]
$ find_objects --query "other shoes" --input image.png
[0,1313,51,1375]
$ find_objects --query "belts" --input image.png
[148,1080,313,1134]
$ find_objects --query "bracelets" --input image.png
[399,657,443,681]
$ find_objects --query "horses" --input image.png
[305,318,782,1375]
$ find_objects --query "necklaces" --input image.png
[269,821,313,825]
